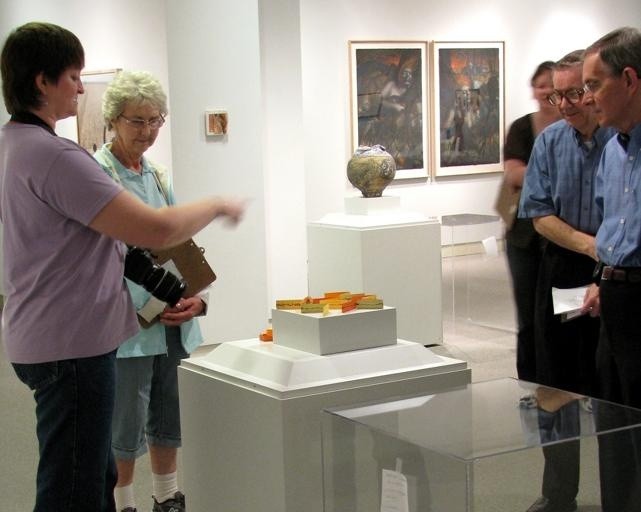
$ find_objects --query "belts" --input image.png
[591,264,641,284]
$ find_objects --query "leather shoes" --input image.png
[523,493,579,512]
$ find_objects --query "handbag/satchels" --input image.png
[496,181,522,232]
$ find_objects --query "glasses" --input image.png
[547,89,585,106]
[583,72,624,94]
[120,113,166,130]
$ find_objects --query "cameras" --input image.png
[118,244,187,308]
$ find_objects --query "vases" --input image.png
[344,146,397,197]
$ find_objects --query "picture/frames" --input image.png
[431,41,506,179]
[348,39,430,180]
[206,111,228,136]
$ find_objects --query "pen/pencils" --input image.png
[585,306,601,317]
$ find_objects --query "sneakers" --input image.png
[151,490,186,511]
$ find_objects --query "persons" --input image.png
[582,26,641,511]
[0,24,253,511]
[92,70,212,511]
[378,64,422,127]
[501,60,554,434]
[440,81,498,162]
[519,52,616,512]
